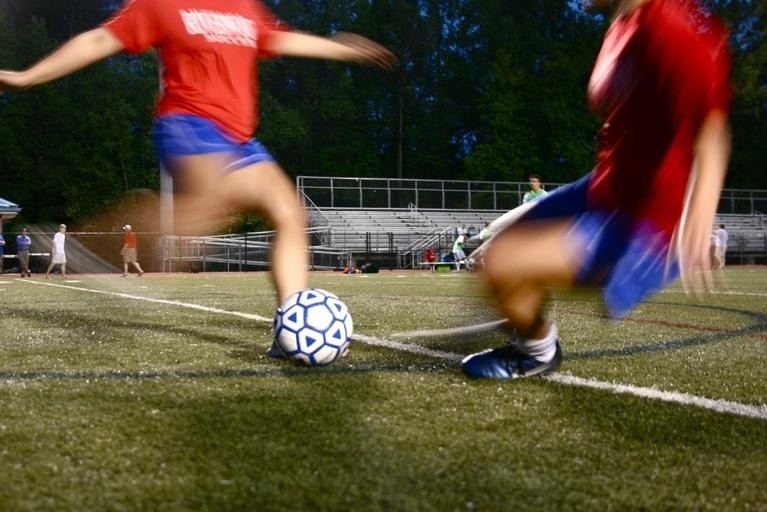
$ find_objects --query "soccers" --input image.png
[469,258,475,265]
[274,288,353,365]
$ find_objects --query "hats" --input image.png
[121,224,132,231]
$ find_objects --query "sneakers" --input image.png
[458,343,563,383]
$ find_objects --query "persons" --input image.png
[451,233,473,272]
[15,226,32,278]
[44,223,70,280]
[118,224,144,278]
[478,222,493,270]
[424,248,436,273]
[457,0,736,381]
[0,3,402,361]
[0,227,6,274]
[520,174,548,205]
[712,224,728,271]
